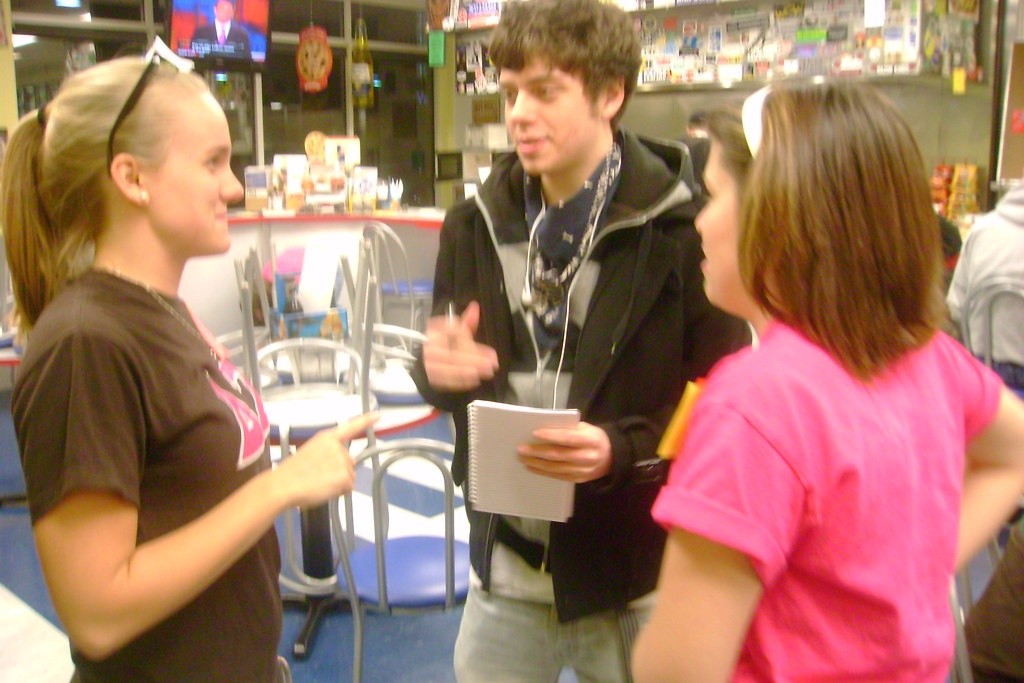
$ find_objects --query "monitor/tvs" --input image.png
[163,0,274,73]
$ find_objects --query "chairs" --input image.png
[329,436,470,683]
[962,276,1024,400]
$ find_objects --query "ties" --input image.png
[218,26,227,46]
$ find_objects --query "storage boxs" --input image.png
[244,135,404,216]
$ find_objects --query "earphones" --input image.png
[520,285,532,310]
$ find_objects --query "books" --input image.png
[465,400,581,522]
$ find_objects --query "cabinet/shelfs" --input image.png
[444,0,989,98]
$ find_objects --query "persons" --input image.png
[0,54,379,683]
[410,0,751,683]
[628,79,1024,683]
[188,0,252,61]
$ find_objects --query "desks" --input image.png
[234,356,442,658]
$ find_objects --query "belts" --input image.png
[494,518,550,571]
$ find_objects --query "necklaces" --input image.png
[101,264,221,360]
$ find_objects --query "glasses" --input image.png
[107,34,197,178]
